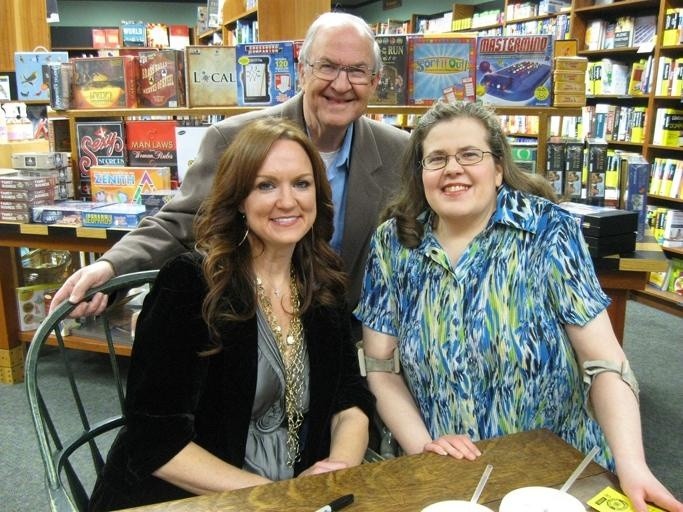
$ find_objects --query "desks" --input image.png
[99,422,681,510]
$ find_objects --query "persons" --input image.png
[351,97,683,511]
[49,11,411,344]
[87,116,377,512]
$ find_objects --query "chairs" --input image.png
[20,269,383,510]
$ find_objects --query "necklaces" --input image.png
[255,266,291,296]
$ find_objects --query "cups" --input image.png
[417,499,497,512]
[498,485,586,512]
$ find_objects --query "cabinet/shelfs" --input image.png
[2,0,682,401]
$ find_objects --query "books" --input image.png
[583,8,683,250]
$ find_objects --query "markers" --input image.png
[315,494,354,512]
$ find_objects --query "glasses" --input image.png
[415,145,500,172]
[300,57,378,86]
